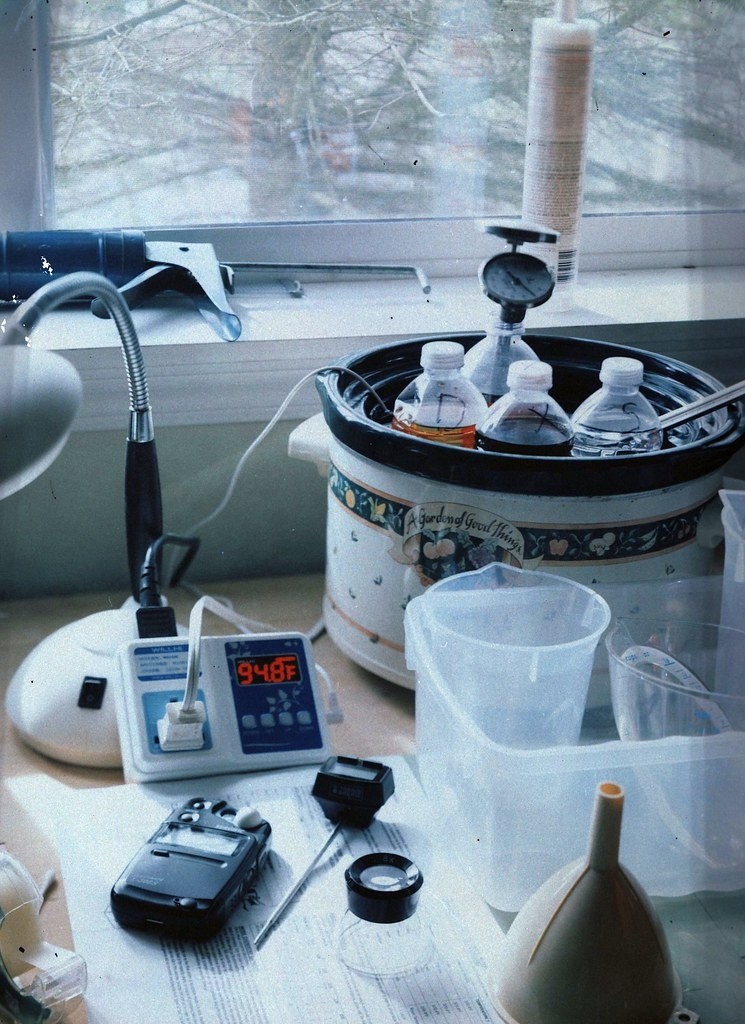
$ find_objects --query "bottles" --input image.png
[474,359,574,458]
[392,341,489,452]
[570,356,663,457]
[462,311,540,408]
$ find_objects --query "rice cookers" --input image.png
[283,331,744,713]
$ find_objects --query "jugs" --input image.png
[423,561,612,754]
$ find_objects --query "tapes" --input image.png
[0,868,78,985]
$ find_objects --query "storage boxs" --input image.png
[402,574,745,914]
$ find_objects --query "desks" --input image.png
[0,573,745,1024]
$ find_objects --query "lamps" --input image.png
[0,271,189,773]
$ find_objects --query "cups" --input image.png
[602,615,745,873]
